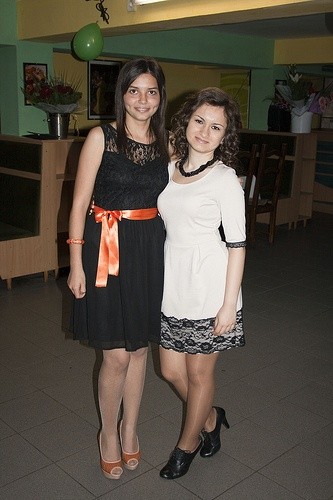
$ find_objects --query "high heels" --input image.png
[160,438,203,479]
[98,430,123,479]
[117,420,140,471]
[199,406,230,457]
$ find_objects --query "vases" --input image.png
[291,112,313,133]
[47,113,70,140]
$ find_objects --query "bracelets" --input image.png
[66,239,85,244]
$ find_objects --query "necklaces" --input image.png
[125,124,153,139]
[178,157,217,177]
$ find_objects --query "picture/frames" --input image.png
[87,59,123,120]
[23,62,48,106]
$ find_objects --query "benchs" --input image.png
[0,128,333,290]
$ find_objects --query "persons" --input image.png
[60,58,168,481]
[156,87,246,479]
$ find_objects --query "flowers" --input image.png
[20,71,83,113]
[262,63,333,115]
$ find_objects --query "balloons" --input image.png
[73,22,104,61]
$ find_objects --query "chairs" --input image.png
[239,144,259,247]
[244,143,288,249]
